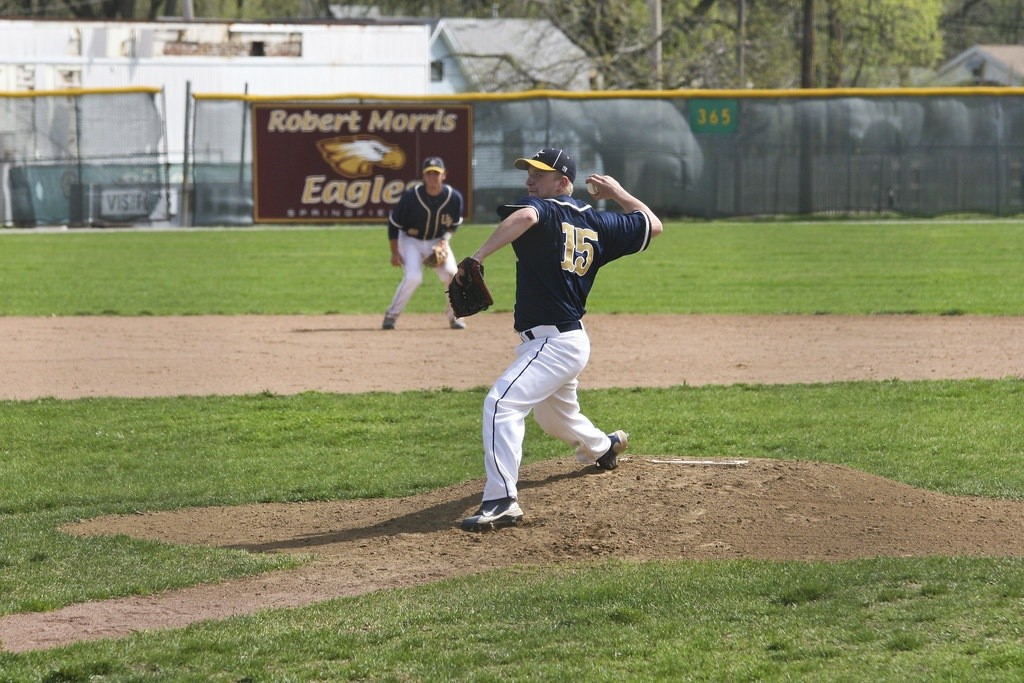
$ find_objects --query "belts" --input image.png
[520,321,582,343]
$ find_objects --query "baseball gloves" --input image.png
[448,257,494,318]
[421,239,449,267]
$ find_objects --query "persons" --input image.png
[446,149,663,528]
[384,155,466,330]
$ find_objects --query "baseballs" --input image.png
[586,182,598,194]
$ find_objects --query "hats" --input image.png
[515,148,576,184]
[422,157,444,174]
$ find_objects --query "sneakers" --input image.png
[596,430,627,470]
[460,497,523,533]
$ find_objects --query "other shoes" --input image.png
[450,318,465,329]
[382,316,395,329]
